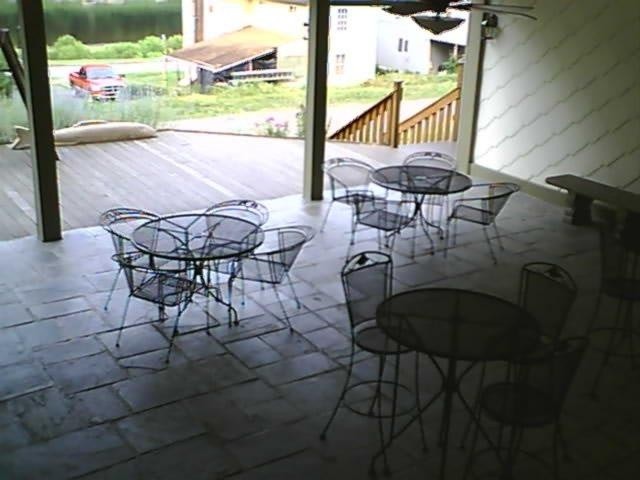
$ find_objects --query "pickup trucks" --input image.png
[70,64,127,101]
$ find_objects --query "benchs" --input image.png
[543,171,638,240]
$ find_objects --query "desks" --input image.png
[367,163,474,256]
[364,286,545,478]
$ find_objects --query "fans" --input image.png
[272,0,538,35]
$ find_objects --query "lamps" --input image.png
[480,11,502,39]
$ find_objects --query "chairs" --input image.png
[322,250,431,475]
[461,335,592,479]
[99,198,317,363]
[320,155,389,245]
[495,260,581,409]
[393,151,458,235]
[584,227,636,367]
[442,179,522,265]
[341,186,426,266]
